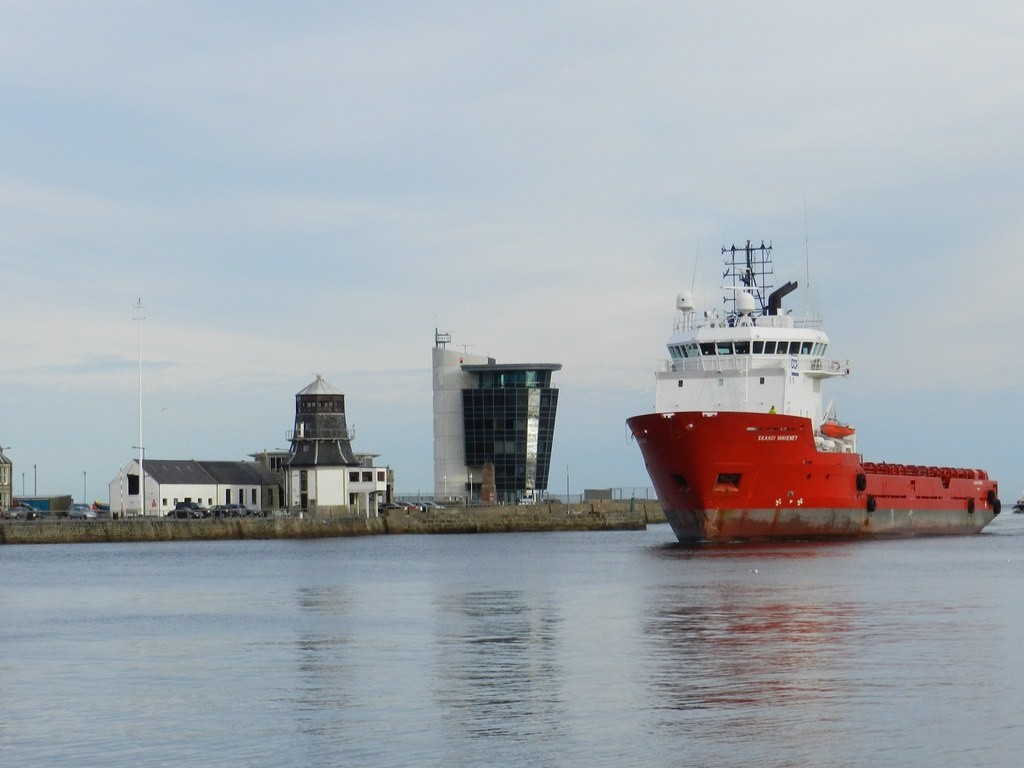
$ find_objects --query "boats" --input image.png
[819,418,854,439]
[1011,498,1024,511]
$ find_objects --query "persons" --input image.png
[769,405,776,414]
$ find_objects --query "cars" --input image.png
[209,505,233,517]
[377,499,446,514]
[226,503,248,517]
[4,506,29,520]
[168,507,201,520]
[22,503,40,520]
[68,507,97,520]
[247,505,264,518]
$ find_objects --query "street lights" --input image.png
[33,463,37,496]
[21,472,25,495]
[132,445,146,517]
[444,473,447,497]
[469,473,473,500]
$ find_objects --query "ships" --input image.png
[624,236,1001,549]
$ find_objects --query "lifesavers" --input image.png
[987,490,1001,514]
[868,497,876,512]
[856,473,867,491]
[968,498,974,513]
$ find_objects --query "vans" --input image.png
[176,501,208,519]
